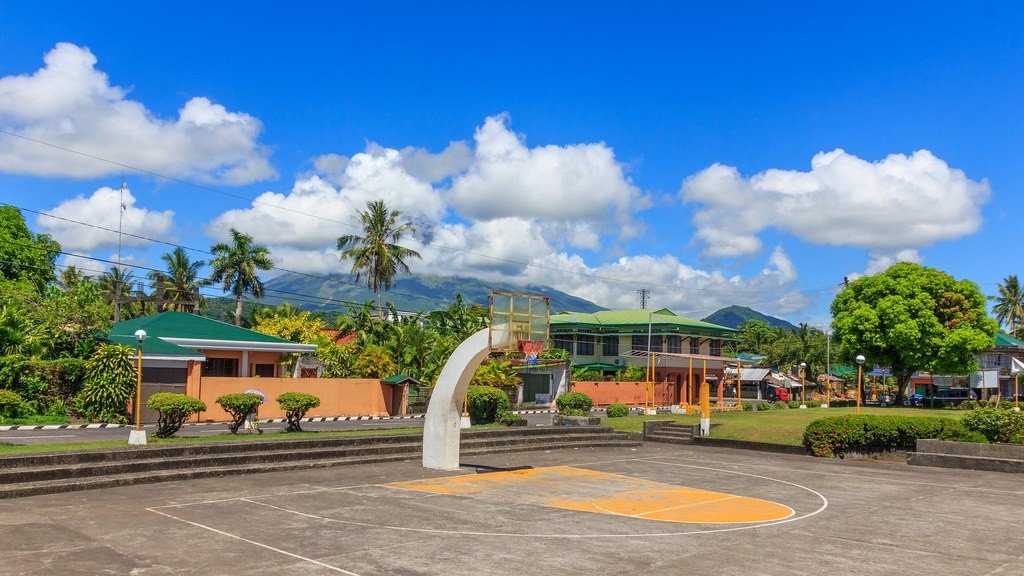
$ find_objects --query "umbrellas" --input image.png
[245,388,269,403]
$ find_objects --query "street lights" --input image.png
[1014,369,1020,408]
[827,331,832,407]
[128,330,147,446]
[800,363,806,409]
[856,355,865,413]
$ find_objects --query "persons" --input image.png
[867,387,874,402]
[908,395,916,406]
[878,394,888,407]
[889,395,895,402]
[244,397,264,435]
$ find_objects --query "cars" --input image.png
[925,388,978,407]
